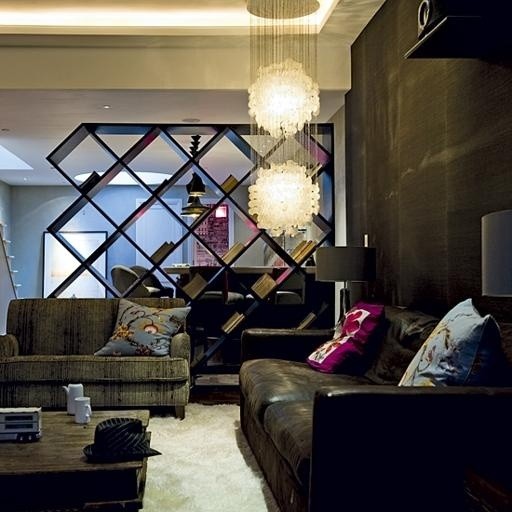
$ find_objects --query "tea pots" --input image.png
[61,383,84,414]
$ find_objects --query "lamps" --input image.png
[247,1,321,233]
[180,133,212,218]
[315,246,377,318]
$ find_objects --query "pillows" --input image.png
[394,296,502,387]
[92,297,192,356]
[306,295,386,375]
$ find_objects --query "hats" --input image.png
[83,418,161,462]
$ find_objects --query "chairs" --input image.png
[110,264,307,304]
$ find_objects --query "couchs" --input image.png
[0,297,191,419]
[238,306,510,512]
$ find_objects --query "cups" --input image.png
[73,396,93,423]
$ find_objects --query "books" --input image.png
[221,310,245,334]
[250,272,278,299]
[149,240,175,264]
[221,173,235,192]
[290,238,318,264]
[220,238,245,269]
[181,273,206,299]
[80,170,102,191]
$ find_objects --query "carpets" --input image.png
[138,401,282,512]
